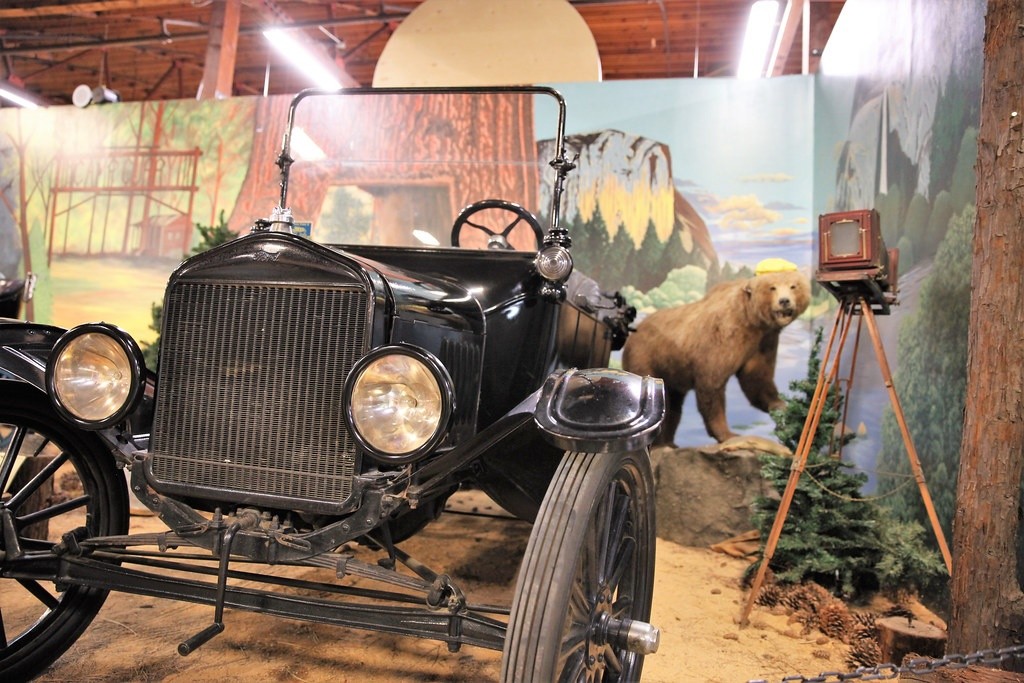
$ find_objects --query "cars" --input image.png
[0,86,669,683]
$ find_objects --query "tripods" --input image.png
[736,276,952,633]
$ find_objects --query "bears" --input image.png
[620,269,811,450]
